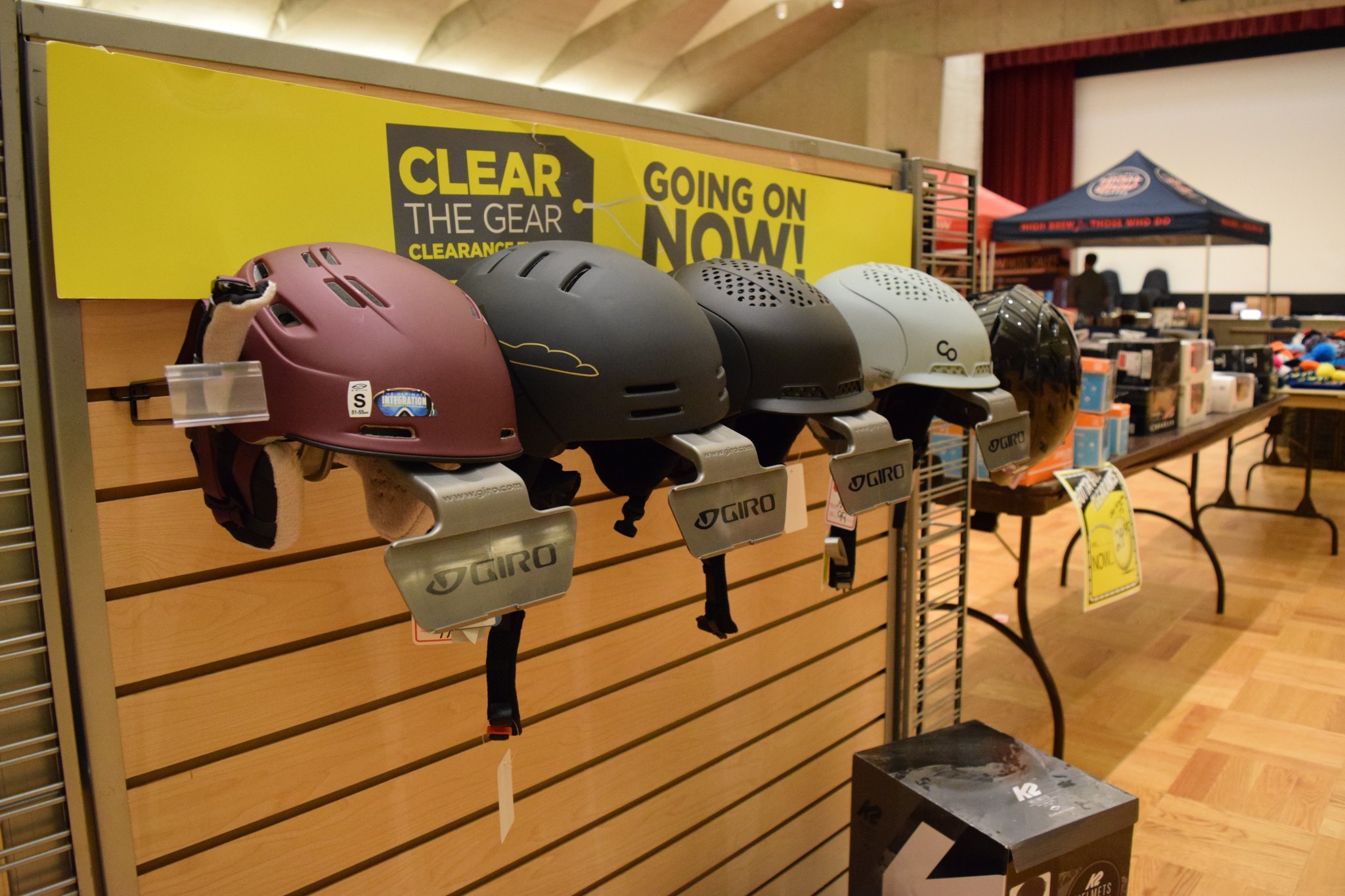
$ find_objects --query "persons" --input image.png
[1078,254,1106,319]
[1052,257,1076,306]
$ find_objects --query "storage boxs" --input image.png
[846,719,1141,896]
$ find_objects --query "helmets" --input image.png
[172,243,524,550]
[812,263,1000,393]
[454,239,732,459]
[665,260,875,416]
[964,284,1082,488]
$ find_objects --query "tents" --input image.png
[921,165,1026,296]
[990,150,1272,340]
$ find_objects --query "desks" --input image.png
[923,395,1297,756]
